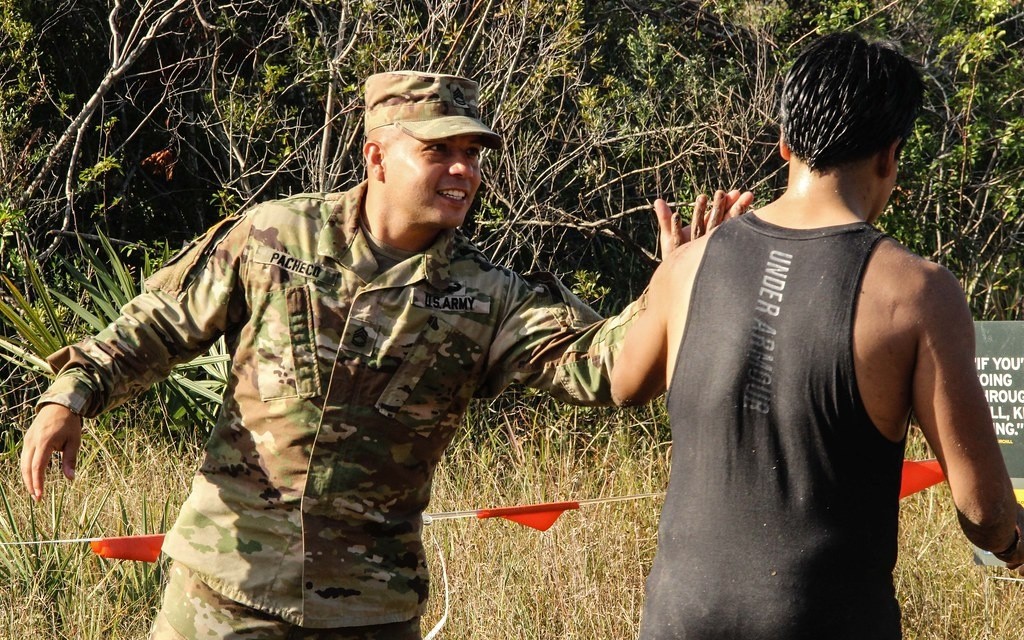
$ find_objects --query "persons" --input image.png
[19,72,738,640]
[608,29,1024,640]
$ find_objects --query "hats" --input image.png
[361,69,508,149]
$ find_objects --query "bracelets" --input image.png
[993,521,1022,559]
